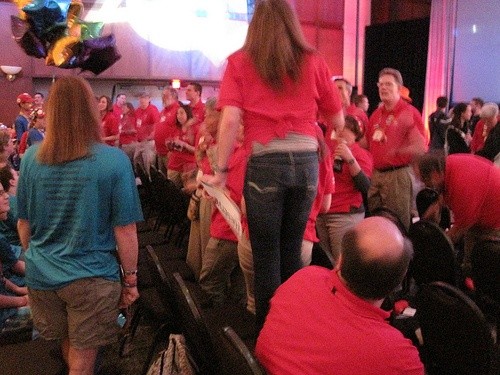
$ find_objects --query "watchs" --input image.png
[346,156,357,167]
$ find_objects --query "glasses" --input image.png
[377,82,399,90]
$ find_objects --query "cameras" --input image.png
[117,310,132,329]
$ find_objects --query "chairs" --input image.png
[116,245,215,361]
[416,187,452,231]
[222,326,263,375]
[368,206,410,242]
[309,242,336,271]
[415,281,500,375]
[408,220,461,289]
[128,244,198,341]
[138,245,260,375]
[469,235,499,328]
[173,273,260,375]
[0,339,70,375]
[131,152,193,262]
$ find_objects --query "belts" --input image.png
[375,166,400,173]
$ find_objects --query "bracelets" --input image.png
[213,163,231,172]
[122,281,138,288]
[123,270,138,276]
[191,192,201,203]
[22,296,29,307]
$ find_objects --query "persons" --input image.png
[254,216,425,375]
[419,150,499,303]
[194,95,247,309]
[238,123,335,315]
[18,75,142,375]
[201,1,346,350]
[299,76,500,271]
[8,84,208,221]
[0,123,35,345]
[358,69,429,234]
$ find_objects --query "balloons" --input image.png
[10,0,121,76]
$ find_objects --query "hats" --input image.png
[16,93,37,104]
[133,91,151,99]
[29,109,47,120]
[400,86,412,102]
